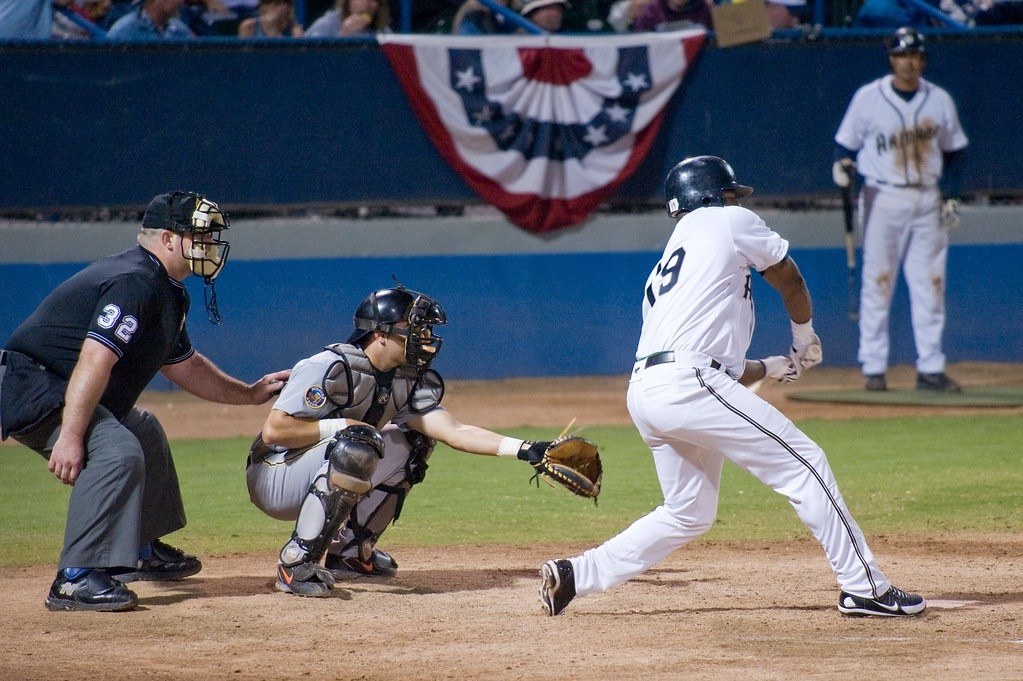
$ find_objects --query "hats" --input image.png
[520,0,571,15]
[771,0,807,17]
[142,190,226,234]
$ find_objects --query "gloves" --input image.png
[831,156,858,187]
[760,354,799,385]
[516,439,558,474]
[941,198,962,231]
[789,317,823,376]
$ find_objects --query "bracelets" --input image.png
[317,417,347,441]
[498,437,524,457]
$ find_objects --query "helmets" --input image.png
[344,289,447,345]
[887,27,926,56]
[665,155,753,217]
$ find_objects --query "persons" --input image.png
[246,283,603,597]
[833,27,968,392]
[0,189,292,612]
[0,0,1023,39]
[538,154,926,617]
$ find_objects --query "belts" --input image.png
[646,350,731,377]
[246,449,278,469]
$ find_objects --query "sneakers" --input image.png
[105,539,204,582]
[325,549,399,578]
[44,567,138,611]
[839,583,926,618]
[275,562,333,599]
[915,372,960,393]
[536,559,576,618]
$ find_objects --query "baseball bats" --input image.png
[747,344,822,392]
[838,159,860,322]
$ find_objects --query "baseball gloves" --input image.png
[529,435,603,507]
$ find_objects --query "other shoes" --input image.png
[866,373,887,390]
